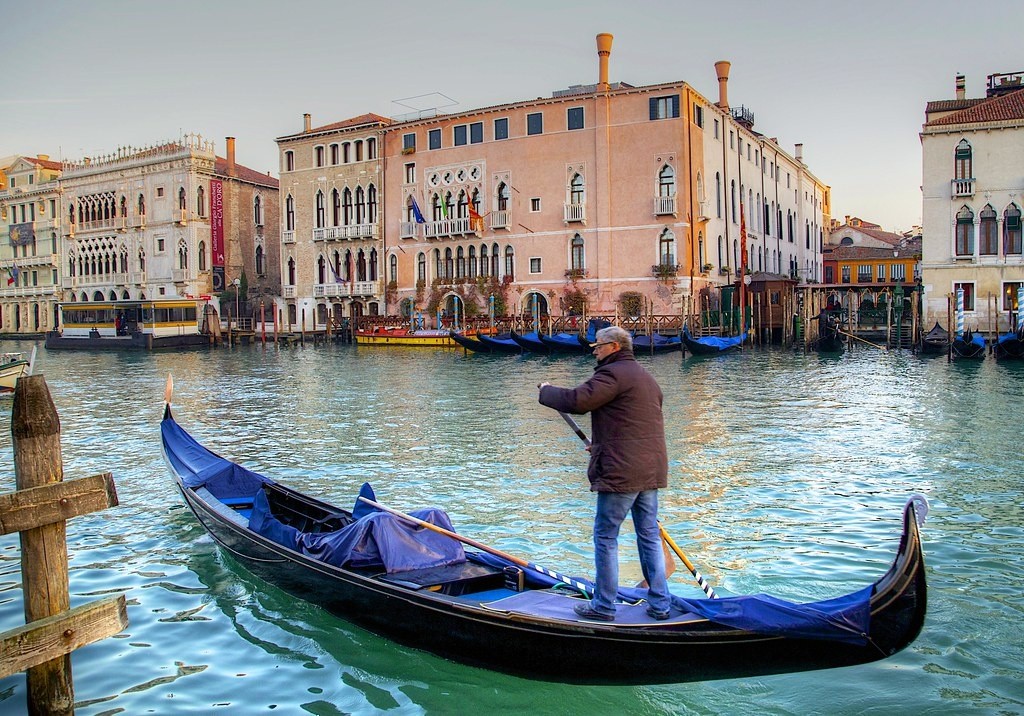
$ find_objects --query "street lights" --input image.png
[233,279,241,329]
[893,280,904,351]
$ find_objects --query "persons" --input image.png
[810,300,843,337]
[538,326,671,619]
[115,313,127,337]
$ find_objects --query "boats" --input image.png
[951,324,986,358]
[577,334,683,354]
[448,329,491,351]
[992,322,1024,361]
[538,319,637,353]
[815,334,844,352]
[159,372,930,687]
[0,352,32,394]
[476,330,539,352]
[680,321,749,354]
[509,327,555,353]
[922,319,949,353]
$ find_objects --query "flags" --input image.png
[441,195,449,220]
[468,192,480,218]
[7,262,20,286]
[410,198,426,224]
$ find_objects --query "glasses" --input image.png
[593,340,614,349]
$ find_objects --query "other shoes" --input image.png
[573,601,615,620]
[645,604,670,619]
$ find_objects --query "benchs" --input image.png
[377,560,508,589]
